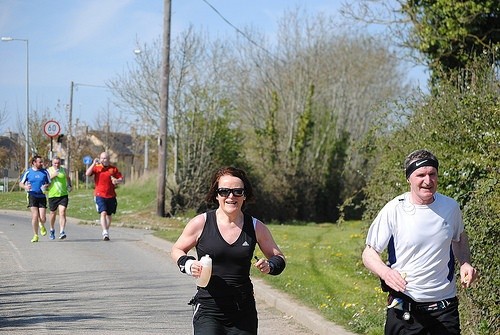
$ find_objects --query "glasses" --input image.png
[215,187,246,197]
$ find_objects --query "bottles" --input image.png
[43,182,49,194]
[110,176,118,187]
[195,254,212,287]
[67,185,71,193]
[27,182,33,191]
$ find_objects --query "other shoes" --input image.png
[40,225,47,237]
[49,229,56,240]
[59,231,66,239]
[102,232,110,241]
[31,235,39,242]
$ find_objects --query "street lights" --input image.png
[1,36,31,173]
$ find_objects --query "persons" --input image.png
[45,156,72,240]
[19,156,51,243]
[362,149,478,335]
[171,167,286,335]
[86,152,123,240]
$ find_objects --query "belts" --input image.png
[385,294,453,313]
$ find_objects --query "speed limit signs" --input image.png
[43,120,62,139]
